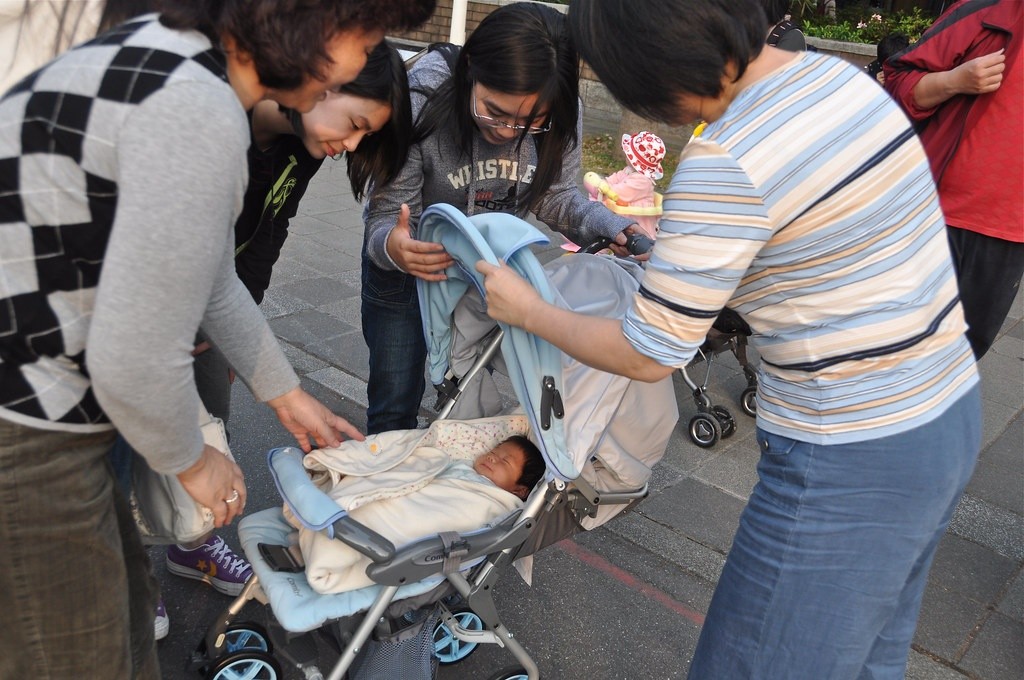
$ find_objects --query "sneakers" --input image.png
[153,595,170,641]
[164,534,254,598]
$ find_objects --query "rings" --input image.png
[227,490,238,502]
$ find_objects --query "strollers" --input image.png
[190,201,679,680]
[578,170,758,450]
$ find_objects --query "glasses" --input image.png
[473,74,553,134]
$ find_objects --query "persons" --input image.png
[602,131,666,237]
[283,435,547,594]
[0,0,437,680]
[868,34,909,87]
[882,0,1024,362]
[361,3,654,436]
[763,0,806,52]
[114,42,415,639]
[476,0,982,680]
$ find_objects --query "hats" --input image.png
[621,130,667,180]
